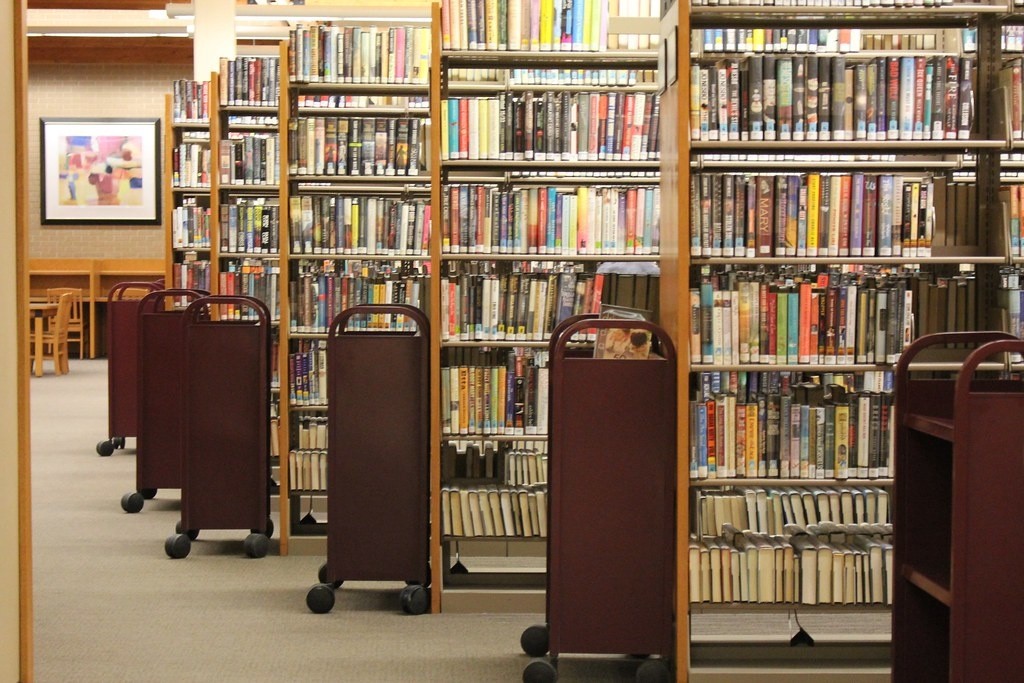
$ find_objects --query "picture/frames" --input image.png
[39,117,161,227]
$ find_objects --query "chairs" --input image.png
[47,288,88,359]
[29,293,72,377]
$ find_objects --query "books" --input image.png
[171,0,1024,606]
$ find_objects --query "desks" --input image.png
[90,257,167,360]
[29,304,57,377]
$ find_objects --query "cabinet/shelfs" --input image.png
[164,0,1024,683]
